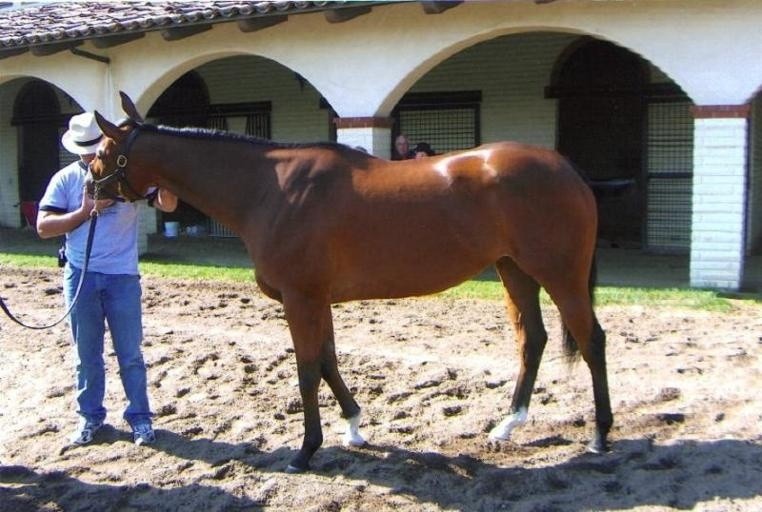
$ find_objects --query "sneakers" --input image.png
[133,423,155,445]
[71,417,103,446]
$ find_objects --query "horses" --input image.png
[83,91,614,474]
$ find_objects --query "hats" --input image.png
[62,113,104,155]
[411,143,434,155]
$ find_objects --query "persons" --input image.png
[36,111,178,446]
[393,133,415,159]
[414,141,432,159]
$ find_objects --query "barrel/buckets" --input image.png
[164,222,179,236]
